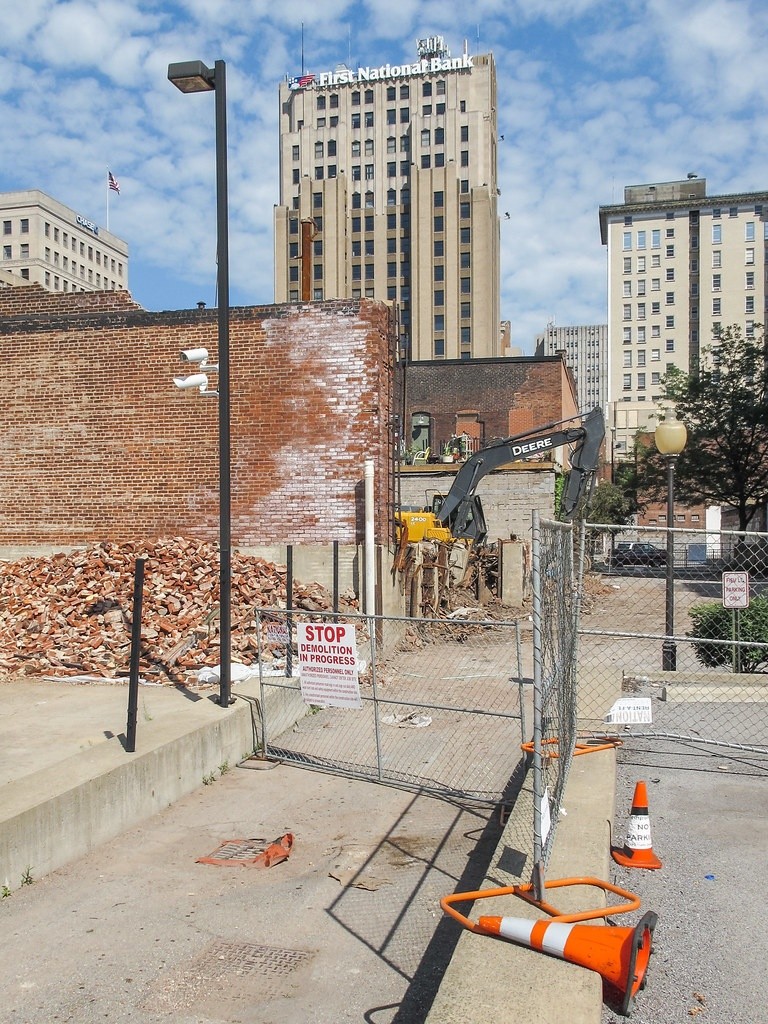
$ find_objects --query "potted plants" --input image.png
[443,441,454,463]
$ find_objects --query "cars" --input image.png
[605,545,674,569]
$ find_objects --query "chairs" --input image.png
[413,447,431,466]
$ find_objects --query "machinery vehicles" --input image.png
[390,405,608,550]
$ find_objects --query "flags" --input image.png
[108,171,120,195]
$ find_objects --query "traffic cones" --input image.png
[477,910,659,1014]
[611,783,667,870]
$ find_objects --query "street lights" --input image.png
[165,56,235,707]
[653,409,690,674]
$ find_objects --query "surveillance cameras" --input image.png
[172,374,208,390]
[179,348,209,362]
